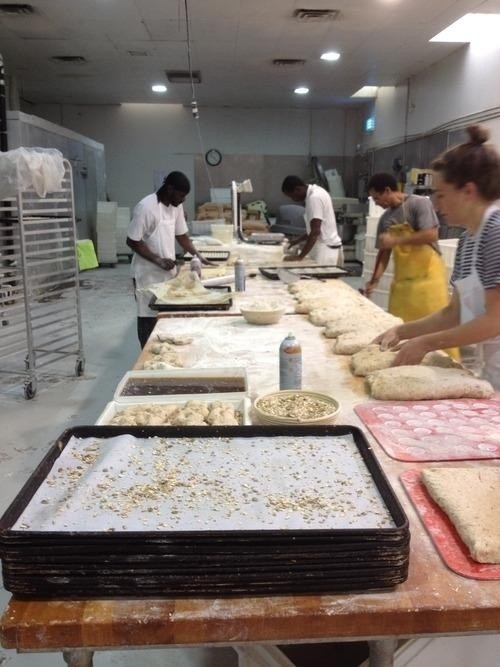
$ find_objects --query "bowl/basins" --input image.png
[240,303,286,325]
[253,390,343,426]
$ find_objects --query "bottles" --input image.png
[191,254,201,280]
[235,257,245,292]
[280,332,302,390]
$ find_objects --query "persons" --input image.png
[374,121,500,397]
[125,170,221,349]
[281,173,345,268]
[363,170,462,364]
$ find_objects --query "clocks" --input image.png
[206,149,222,166]
